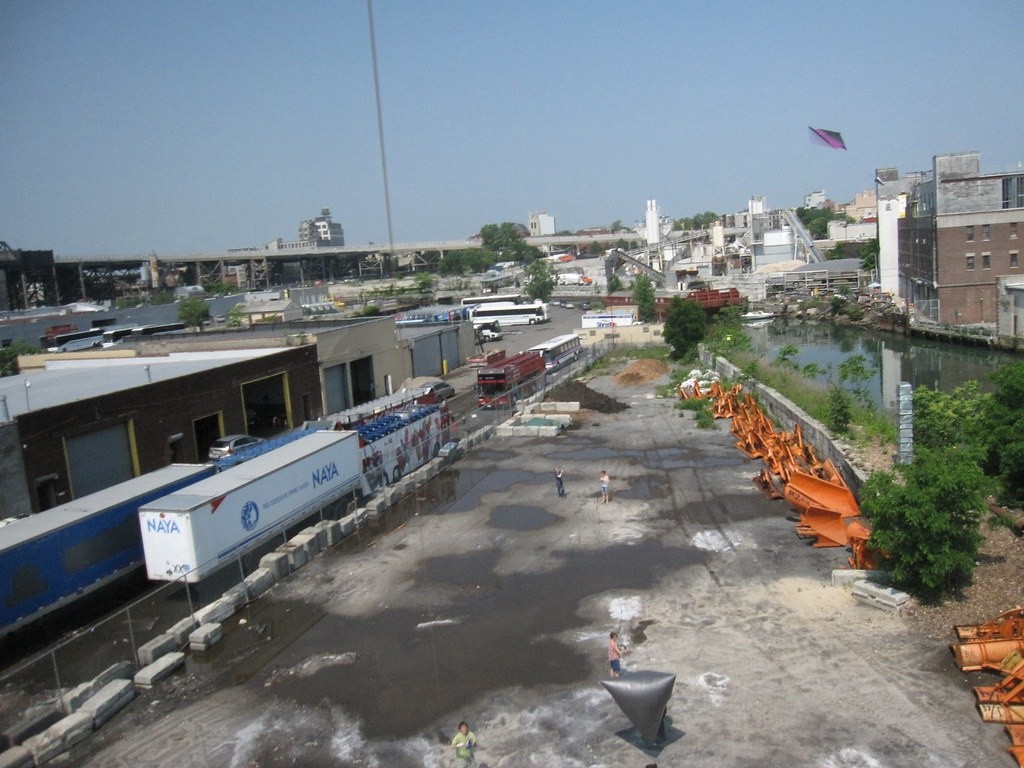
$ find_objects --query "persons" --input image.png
[576,471,598,504]
[554,467,564,498]
[608,631,632,678]
[451,722,478,768]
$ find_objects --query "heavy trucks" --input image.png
[0,388,455,640]
[581,313,643,328]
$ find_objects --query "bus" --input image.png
[474,351,547,410]
[47,327,104,353]
[103,322,186,349]
[526,334,582,371]
[473,320,504,344]
[461,295,550,327]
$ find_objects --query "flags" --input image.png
[816,129,847,149]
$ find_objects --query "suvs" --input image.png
[208,434,265,461]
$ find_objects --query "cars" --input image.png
[419,382,455,403]
